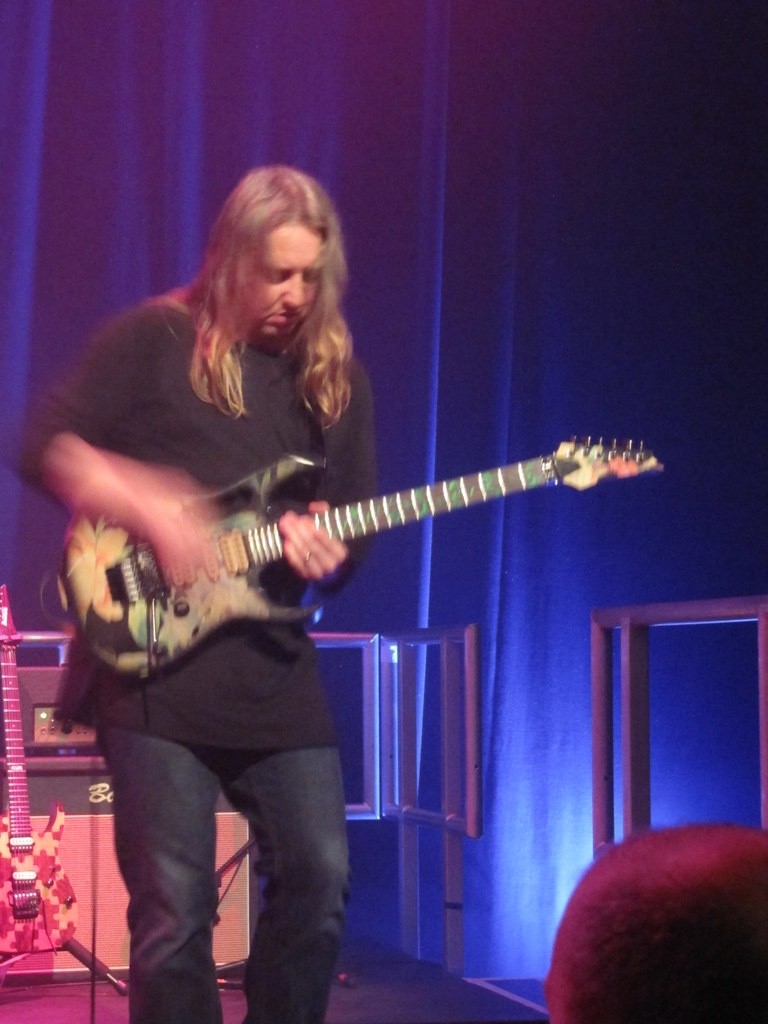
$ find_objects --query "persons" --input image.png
[545,825,768,1024]
[20,162,375,1023]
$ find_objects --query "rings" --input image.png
[303,550,311,565]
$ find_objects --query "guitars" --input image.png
[61,433,666,672]
[0,585,81,959]
